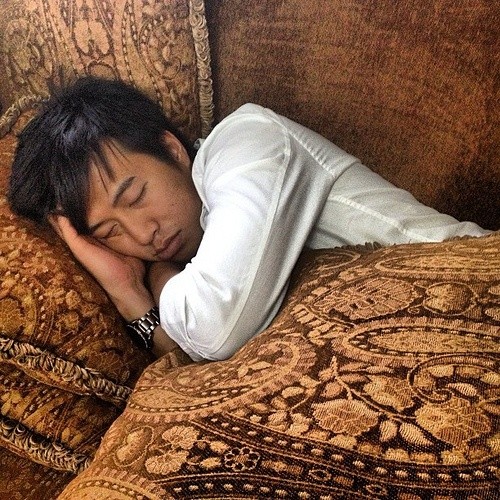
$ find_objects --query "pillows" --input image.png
[0,97,154,473]
[58,231,500,500]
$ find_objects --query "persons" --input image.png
[6,77,494,362]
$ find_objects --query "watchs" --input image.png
[126,306,160,352]
